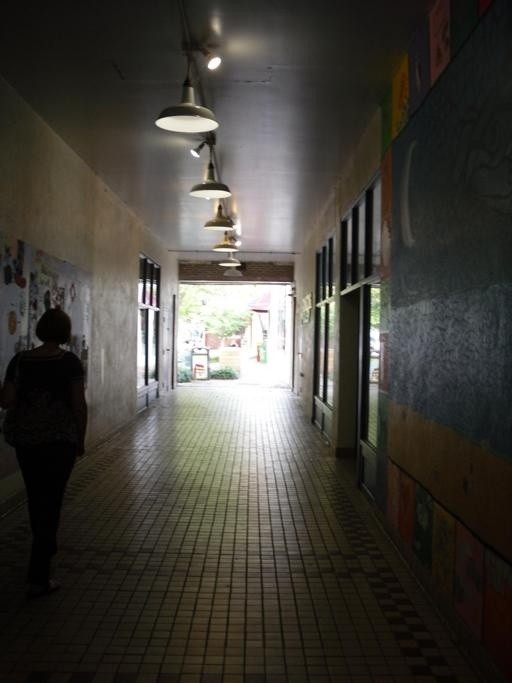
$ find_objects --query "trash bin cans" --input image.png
[191,348,209,380]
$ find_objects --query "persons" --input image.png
[1,307,89,591]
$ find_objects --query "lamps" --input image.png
[153,0,242,282]
